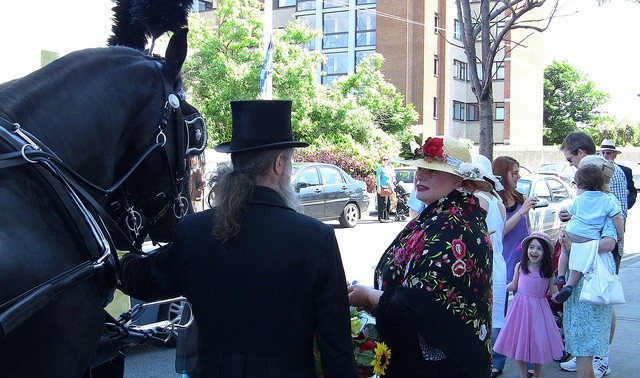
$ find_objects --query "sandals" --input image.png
[555,285,572,302]
[552,276,566,285]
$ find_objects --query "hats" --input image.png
[520,231,554,255]
[471,152,506,191]
[214,99,310,153]
[399,134,493,192]
[596,138,622,155]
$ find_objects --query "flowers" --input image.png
[314,280,392,377]
[400,134,484,181]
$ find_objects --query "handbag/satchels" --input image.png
[579,271,626,305]
[380,188,392,196]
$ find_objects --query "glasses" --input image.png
[601,151,614,155]
[383,158,389,161]
[566,150,578,163]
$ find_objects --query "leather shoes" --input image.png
[491,368,502,377]
[527,369,535,377]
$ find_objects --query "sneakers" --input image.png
[592,356,610,378]
[559,356,577,372]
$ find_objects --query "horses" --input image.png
[0,26,207,377]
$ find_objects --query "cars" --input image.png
[536,163,577,190]
[288,162,372,228]
[374,165,420,212]
[518,174,576,243]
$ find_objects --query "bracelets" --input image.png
[516,210,524,218]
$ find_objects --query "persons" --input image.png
[595,139,637,277]
[553,165,624,304]
[558,155,617,378]
[375,156,395,222]
[118,99,356,378]
[469,153,508,375]
[491,156,538,318]
[188,158,206,213]
[347,136,494,376]
[492,233,564,377]
[559,132,627,377]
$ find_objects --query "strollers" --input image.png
[394,179,413,220]
